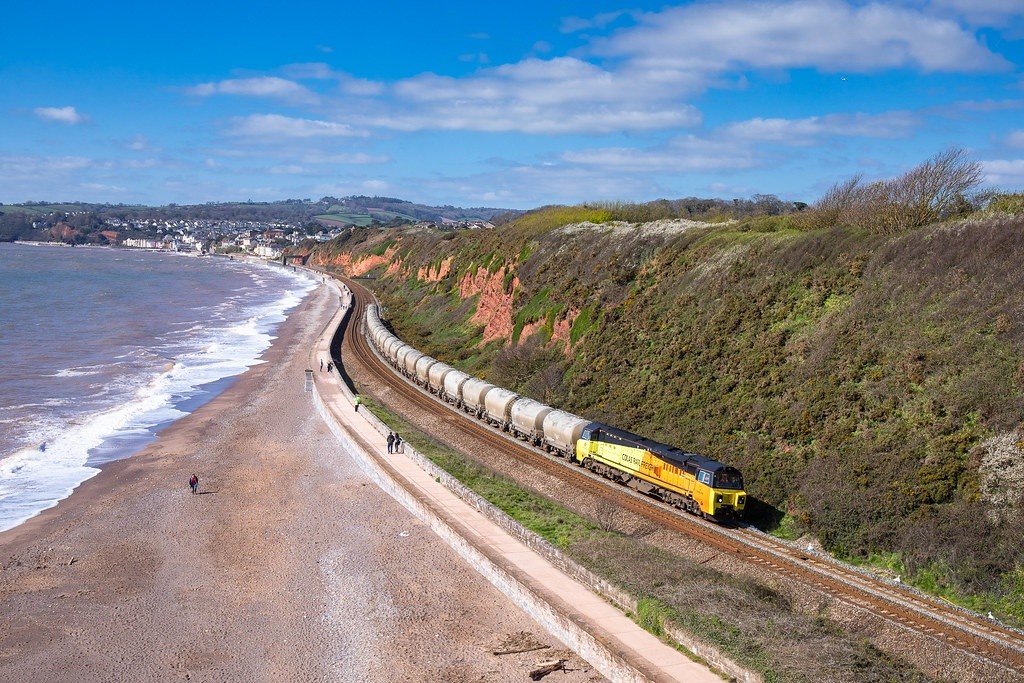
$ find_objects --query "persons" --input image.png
[343,285,345,289]
[340,303,348,309]
[319,358,333,373]
[347,291,350,298]
[353,393,362,412]
[387,431,394,454]
[719,473,738,487]
[393,432,402,452]
[189,473,198,494]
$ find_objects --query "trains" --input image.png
[368,302,747,526]
